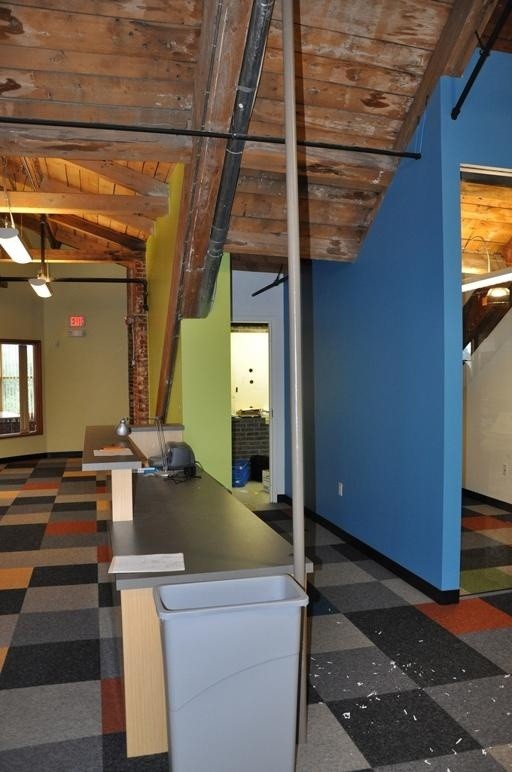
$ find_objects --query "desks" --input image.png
[81,423,315,759]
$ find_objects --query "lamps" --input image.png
[463,236,511,308]
[115,416,174,478]
[0,179,54,298]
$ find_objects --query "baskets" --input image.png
[232,459,249,487]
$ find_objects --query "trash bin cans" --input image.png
[153,574,309,772]
